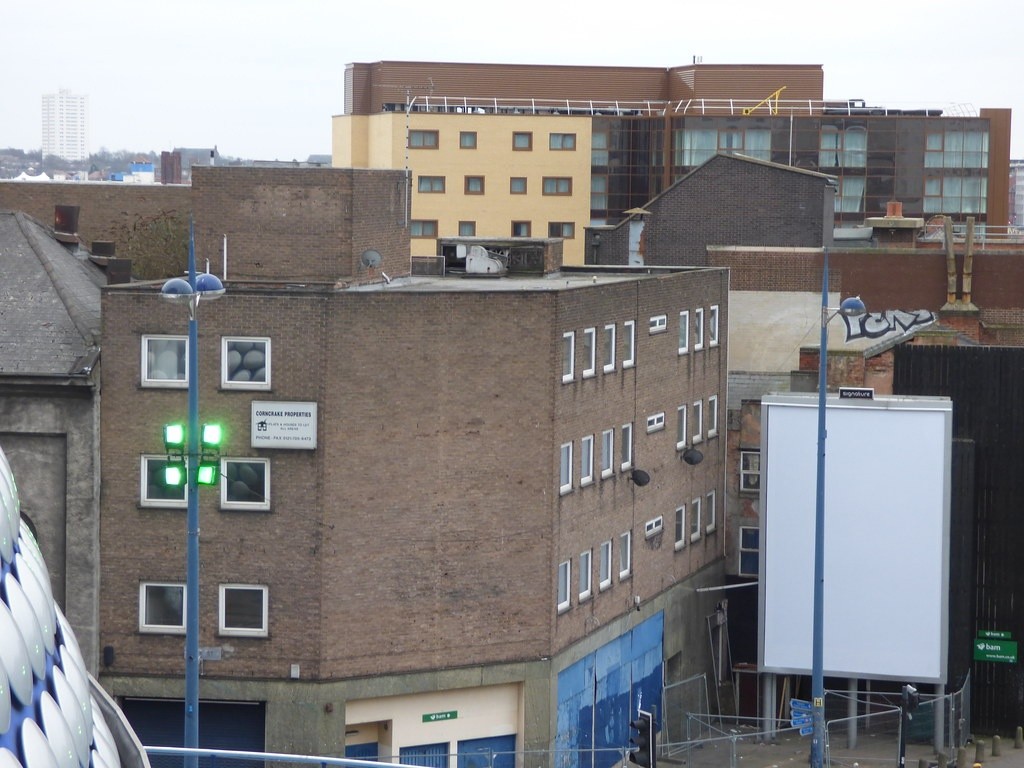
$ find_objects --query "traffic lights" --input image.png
[629,710,656,768]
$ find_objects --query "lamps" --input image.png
[627,470,650,487]
[681,448,704,466]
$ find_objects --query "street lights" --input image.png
[811,297,869,768]
[159,271,225,768]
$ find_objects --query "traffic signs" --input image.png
[789,698,813,736]
